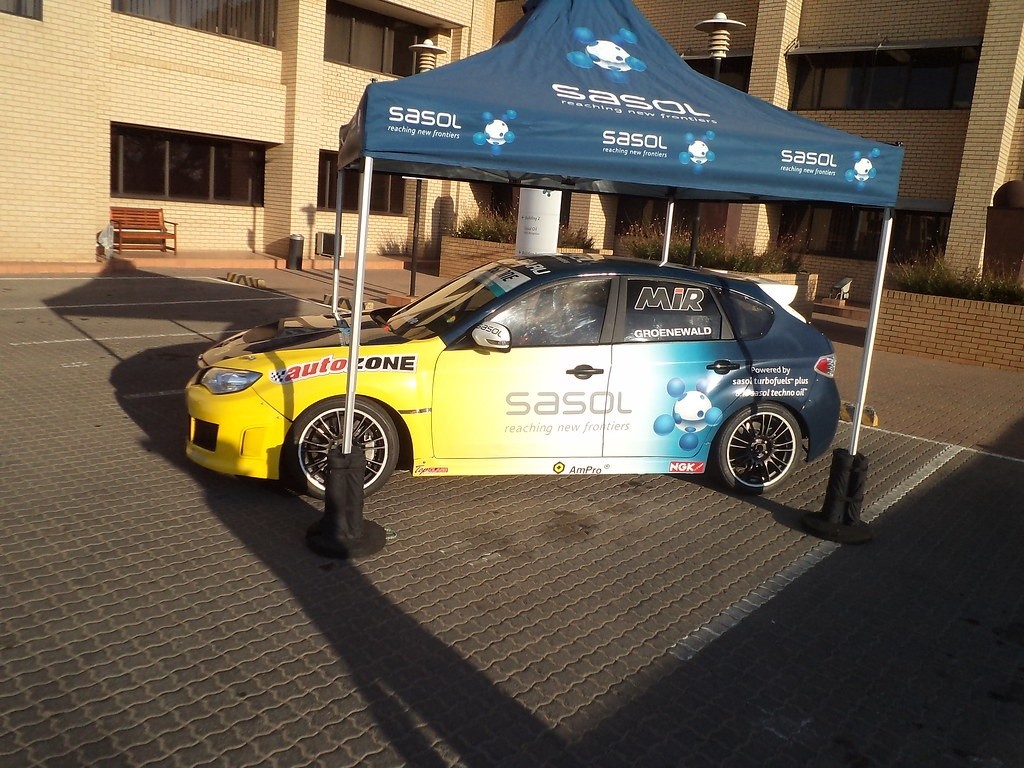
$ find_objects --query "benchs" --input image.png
[109,206,178,256]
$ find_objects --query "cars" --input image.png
[184,254,841,499]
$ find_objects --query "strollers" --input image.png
[829,277,853,300]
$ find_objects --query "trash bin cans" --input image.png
[287,234,305,271]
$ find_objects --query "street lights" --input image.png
[688,12,746,267]
[408,38,447,297]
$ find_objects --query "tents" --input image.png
[304,0,905,560]
